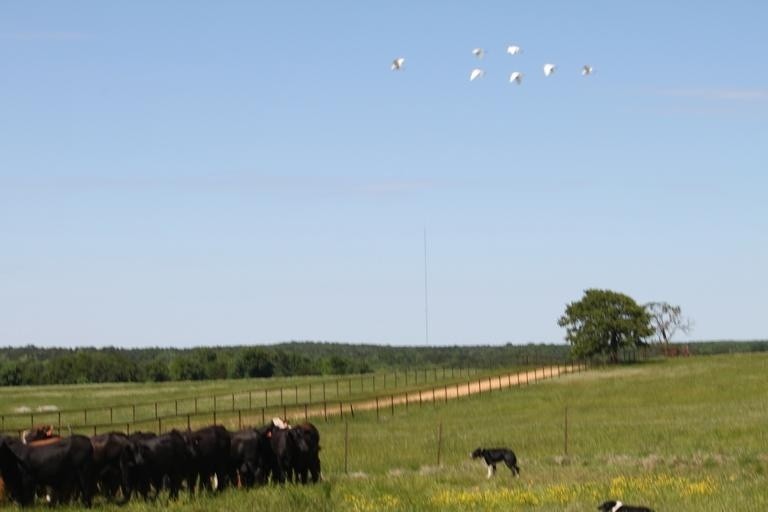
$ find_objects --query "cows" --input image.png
[0,415,323,507]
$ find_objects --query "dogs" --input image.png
[469,447,520,478]
[598,500,653,512]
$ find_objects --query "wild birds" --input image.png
[390,57,404,69]
[469,45,594,85]
